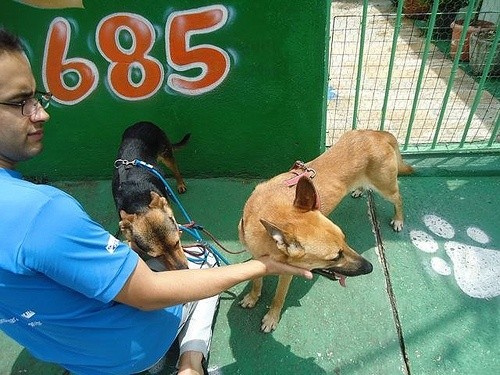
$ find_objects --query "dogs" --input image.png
[112,120,191,271]
[239,129,415,332]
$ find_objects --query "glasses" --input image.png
[0,90,53,116]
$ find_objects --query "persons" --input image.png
[0,32,313,375]
